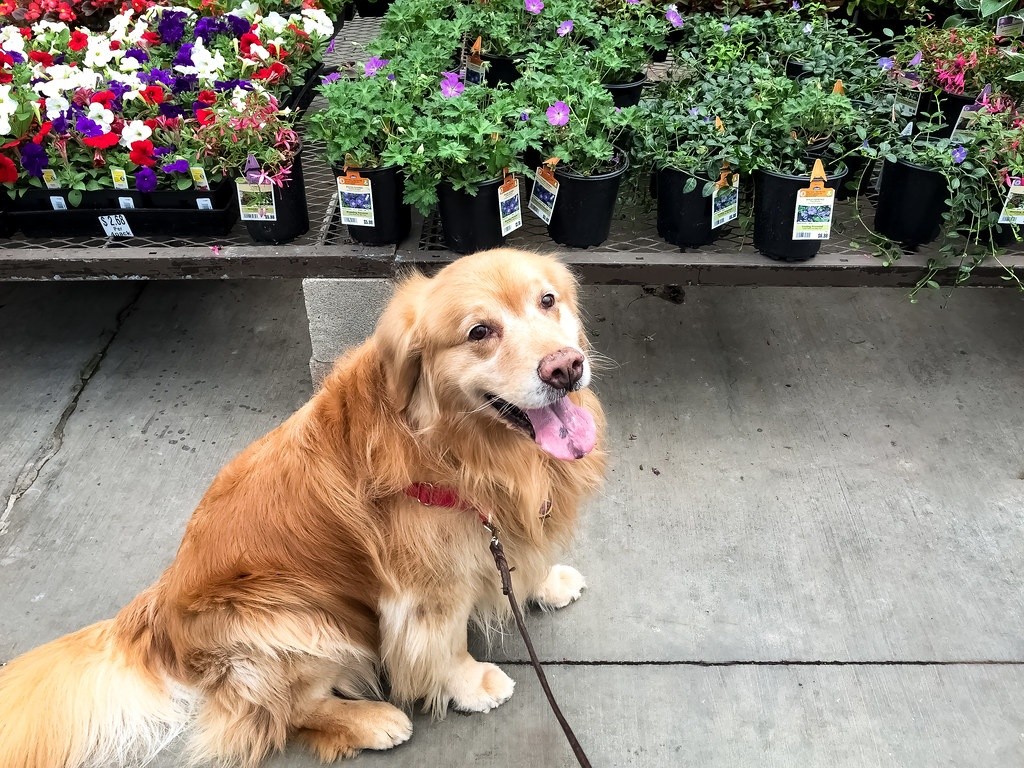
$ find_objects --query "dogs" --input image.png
[1,247,621,768]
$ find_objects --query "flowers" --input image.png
[306,0,1024,312]
[0,0,348,201]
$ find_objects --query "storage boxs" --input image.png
[0,64,325,238]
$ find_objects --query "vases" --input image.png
[650,127,691,198]
[795,70,856,85]
[524,140,555,199]
[786,54,808,77]
[541,143,630,247]
[480,46,533,91]
[956,162,1016,246]
[605,125,632,148]
[834,99,892,200]
[428,158,506,254]
[875,134,962,246]
[918,75,977,138]
[754,151,849,260]
[329,136,413,247]
[231,138,311,245]
[658,161,733,247]
[602,68,647,107]
[0,173,230,210]
[697,55,729,100]
[444,56,463,77]
[355,0,389,17]
[648,40,671,62]
